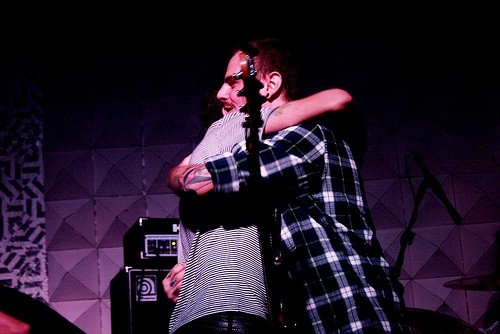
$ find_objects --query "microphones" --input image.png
[413,153,464,225]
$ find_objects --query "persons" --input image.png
[169,40,353,332]
[162,37,417,334]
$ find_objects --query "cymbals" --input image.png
[406,307,481,334]
[443,275,499,291]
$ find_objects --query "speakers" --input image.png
[110,216,182,334]
